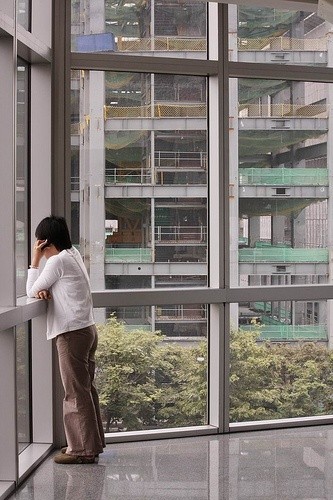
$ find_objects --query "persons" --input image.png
[25,217,107,465]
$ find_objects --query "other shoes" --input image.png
[54,446,99,464]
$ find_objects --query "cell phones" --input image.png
[42,240,51,250]
